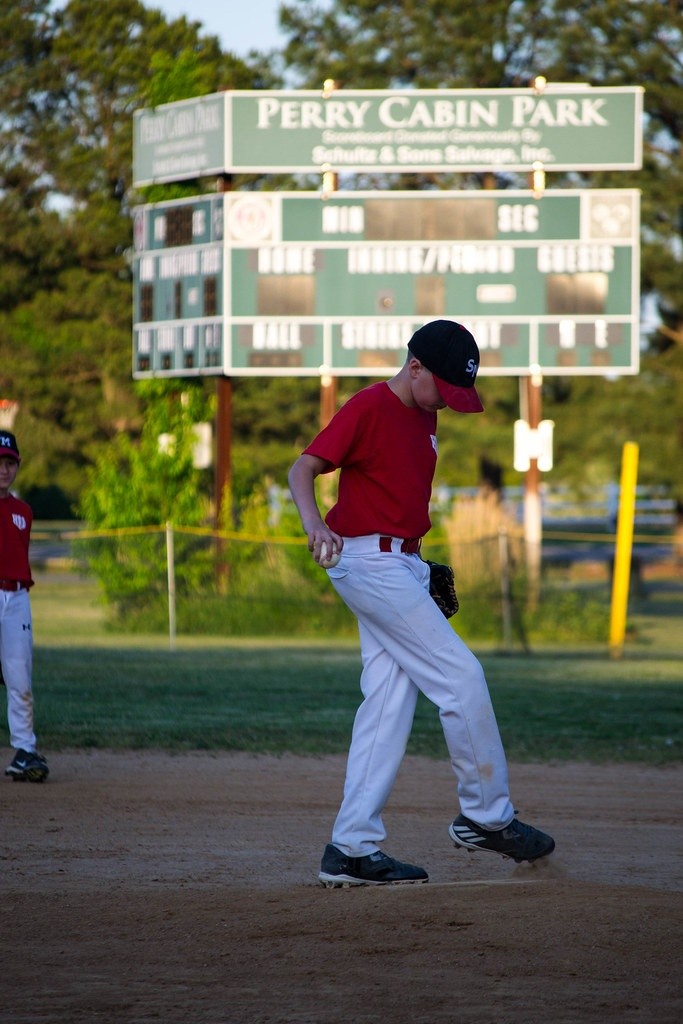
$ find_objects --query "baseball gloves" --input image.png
[423,560,460,619]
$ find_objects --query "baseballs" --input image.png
[312,540,342,570]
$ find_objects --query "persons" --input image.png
[286,320,558,890]
[1,428,50,781]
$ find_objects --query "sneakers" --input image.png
[5,748,48,783]
[318,842,429,890]
[449,810,557,864]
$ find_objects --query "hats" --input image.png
[408,319,486,414]
[0,429,20,460]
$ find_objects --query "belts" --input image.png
[380,538,422,556]
[0,579,35,591]
